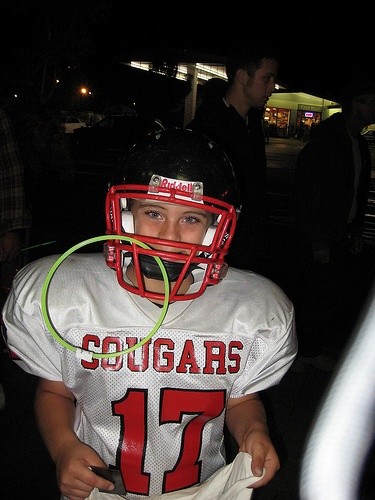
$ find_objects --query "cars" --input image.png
[61,115,87,134]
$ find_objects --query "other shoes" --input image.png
[300,354,336,371]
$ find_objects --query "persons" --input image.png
[0,41,374,409]
[0,129,298,500]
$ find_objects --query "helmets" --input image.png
[104,127,243,302]
